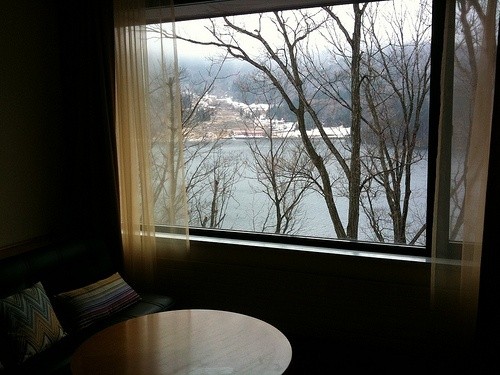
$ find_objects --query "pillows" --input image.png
[55,272,142,332]
[1,280,68,368]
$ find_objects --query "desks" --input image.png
[69,308,292,375]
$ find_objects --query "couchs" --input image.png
[0,228,172,375]
